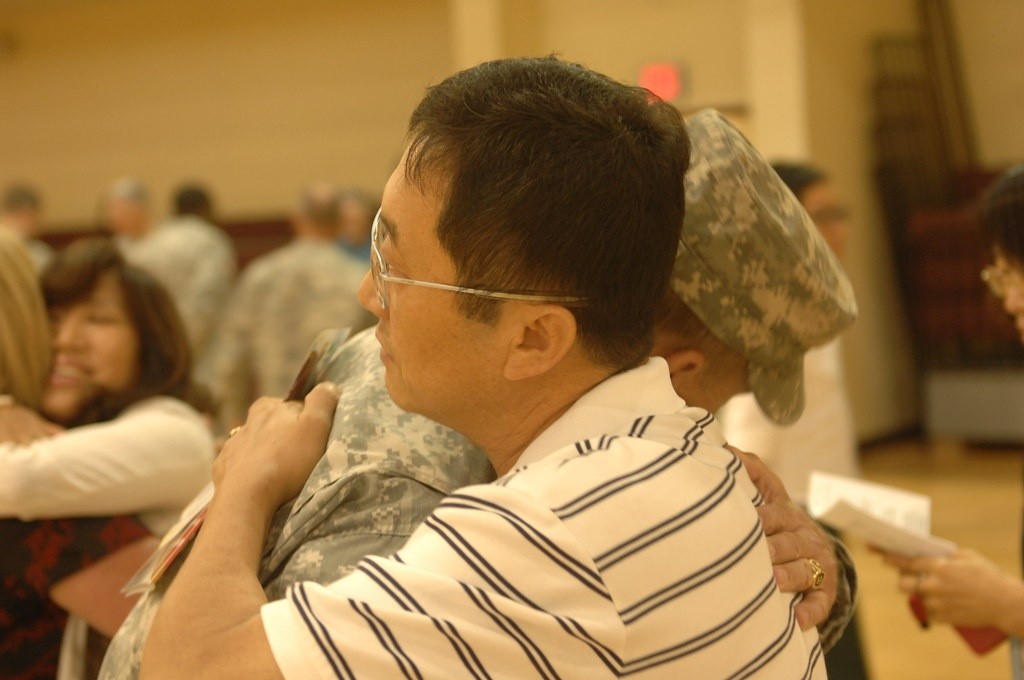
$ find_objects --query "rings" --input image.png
[227,426,240,436]
[809,558,825,590]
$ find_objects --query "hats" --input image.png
[669,107,859,426]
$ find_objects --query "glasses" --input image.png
[369,206,590,310]
[981,264,1018,297]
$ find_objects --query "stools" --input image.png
[923,363,1022,473]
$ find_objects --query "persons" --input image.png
[717,158,872,680]
[211,179,380,435]
[1,177,216,680]
[880,164,1024,679]
[97,106,858,680]
[140,56,827,680]
[129,181,237,354]
[331,188,377,273]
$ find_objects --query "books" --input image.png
[120,482,216,598]
[806,471,958,557]
